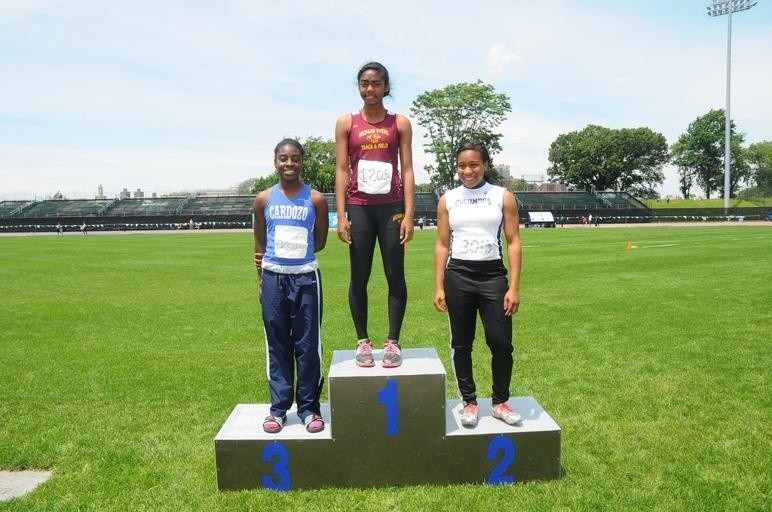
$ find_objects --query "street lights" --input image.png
[706,0,758,218]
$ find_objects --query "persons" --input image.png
[581,216,587,226]
[588,213,592,224]
[434,140,522,427]
[56,221,63,237]
[334,62,416,368]
[189,217,194,230]
[81,221,87,236]
[418,215,424,230]
[253,137,331,433]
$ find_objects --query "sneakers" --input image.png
[355,338,375,366]
[491,402,521,425]
[382,340,402,367]
[461,404,479,426]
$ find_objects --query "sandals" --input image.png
[263,414,287,433]
[305,415,324,433]
